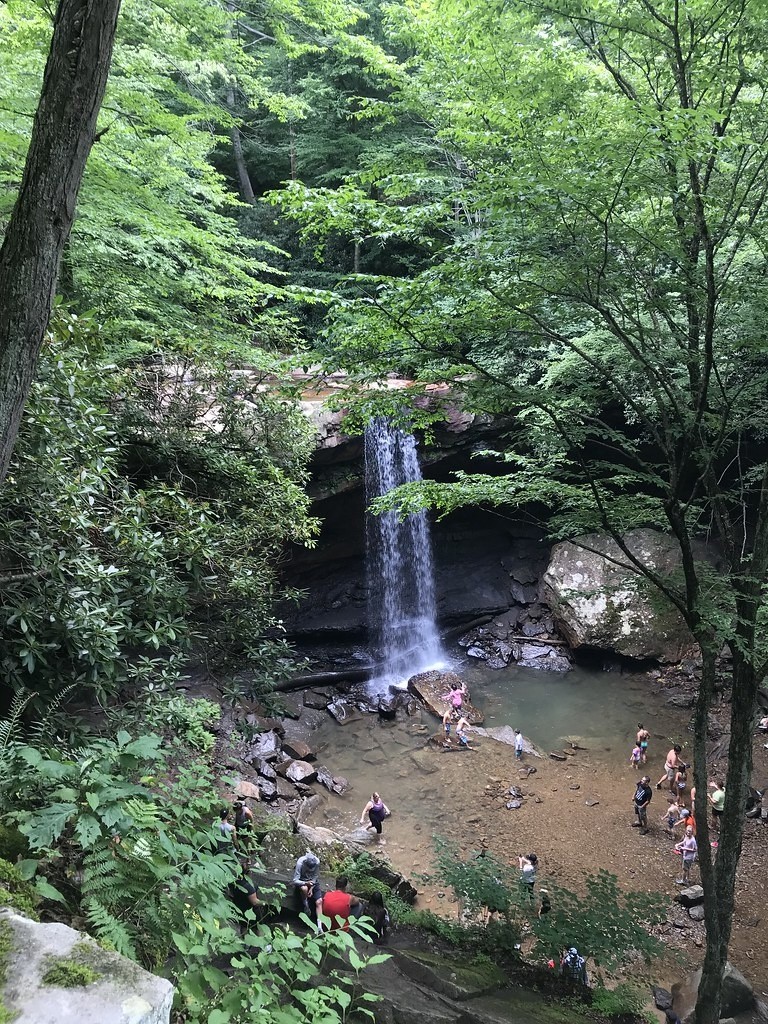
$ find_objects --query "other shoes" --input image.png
[676,879,687,886]
[632,823,643,827]
[655,784,661,789]
[640,828,649,836]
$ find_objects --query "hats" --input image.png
[303,853,318,871]
[679,809,689,819]
[526,853,537,862]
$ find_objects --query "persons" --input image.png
[293,854,392,944]
[637,722,650,763]
[440,683,465,717]
[514,728,524,761]
[707,781,726,829]
[674,809,696,855]
[691,787,696,810]
[233,801,253,842]
[519,853,551,923]
[656,744,687,807]
[675,824,698,885]
[361,791,390,846]
[229,861,265,939]
[560,948,589,992]
[456,715,472,749]
[662,798,680,830]
[630,741,641,770]
[443,708,455,742]
[631,775,652,835]
[211,807,240,857]
